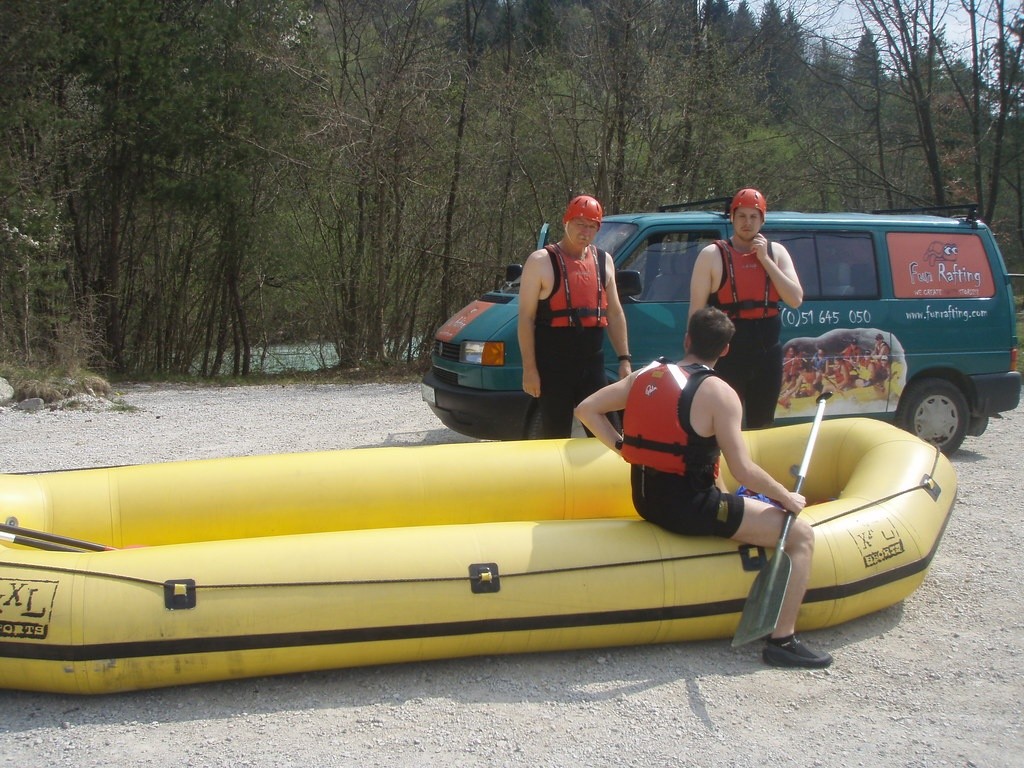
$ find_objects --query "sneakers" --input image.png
[763,633,833,668]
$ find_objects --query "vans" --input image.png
[419,195,1023,459]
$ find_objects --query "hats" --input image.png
[874,334,883,340]
[800,360,811,367]
[833,353,843,361]
[851,338,858,345]
[881,355,889,360]
[865,350,871,355]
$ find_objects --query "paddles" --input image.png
[777,354,857,409]
[730,390,834,648]
[0,522,121,553]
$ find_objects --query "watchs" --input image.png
[618,354,632,363]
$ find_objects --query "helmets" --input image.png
[730,189,766,226]
[563,195,602,232]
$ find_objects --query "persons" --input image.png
[516,195,630,439]
[688,189,803,430]
[575,307,834,669]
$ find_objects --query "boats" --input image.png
[0,414,958,698]
